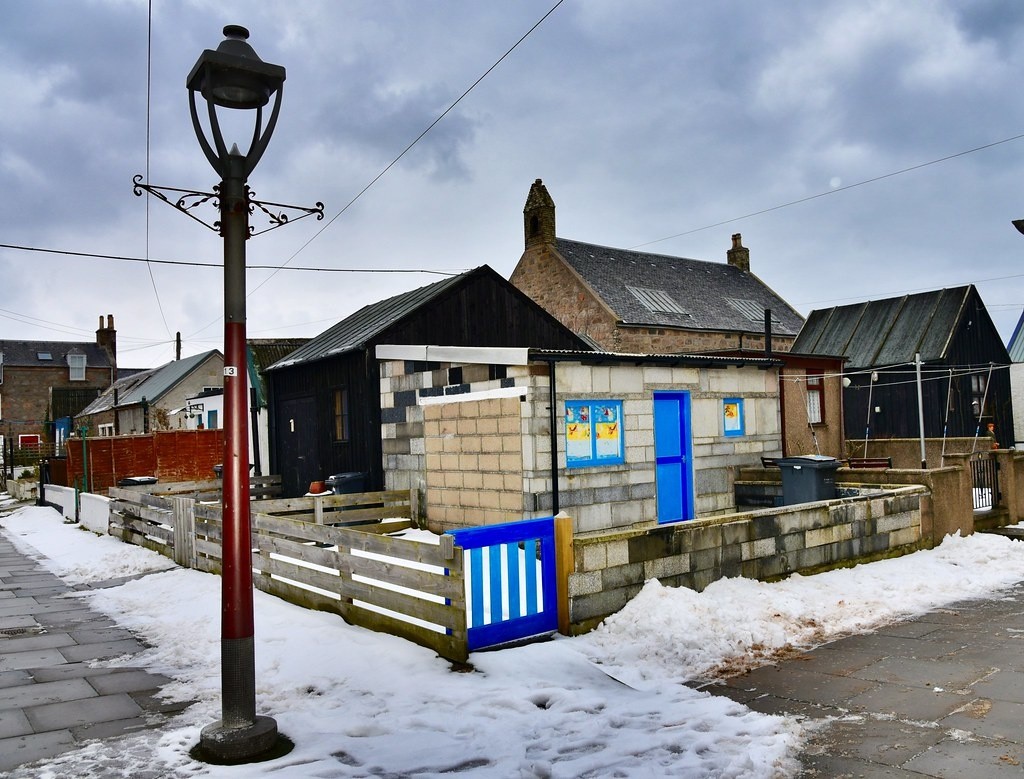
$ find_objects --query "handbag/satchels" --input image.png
[991,443,997,449]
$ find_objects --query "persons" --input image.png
[987,424,999,448]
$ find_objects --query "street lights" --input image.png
[131,23,325,759]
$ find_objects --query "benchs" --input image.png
[847,456,893,469]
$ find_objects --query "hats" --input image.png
[988,424,994,427]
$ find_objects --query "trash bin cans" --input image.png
[213,464,254,478]
[325,472,365,527]
[776,455,842,506]
[121,477,159,486]
